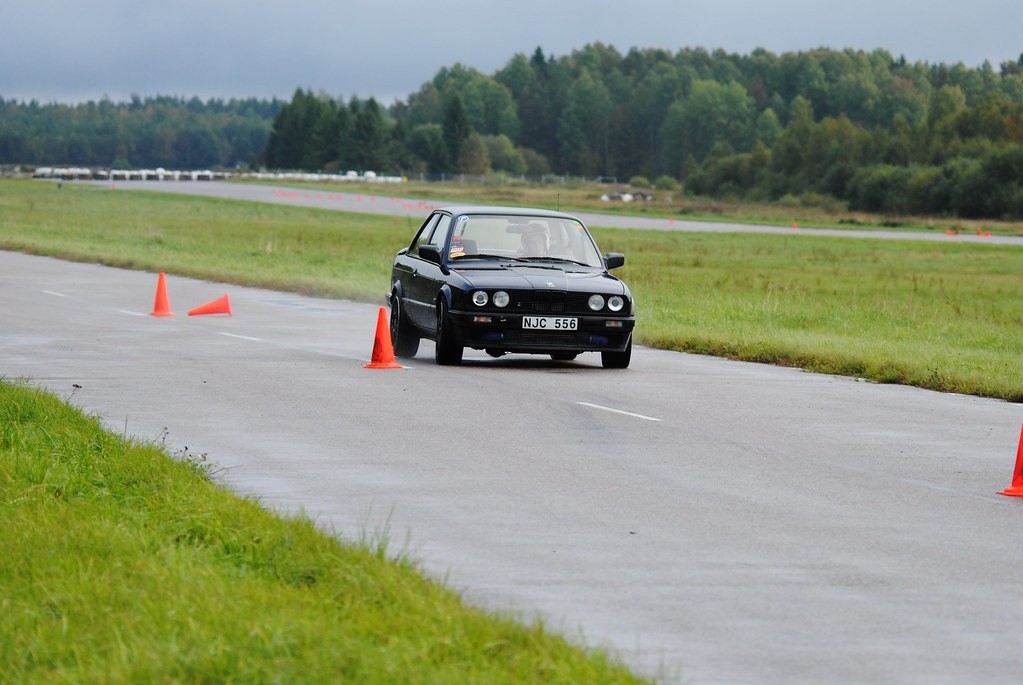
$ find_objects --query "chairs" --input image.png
[452,239,478,256]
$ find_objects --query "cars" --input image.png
[383,205,636,369]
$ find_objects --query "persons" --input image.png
[521,223,551,257]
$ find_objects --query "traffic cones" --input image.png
[995,422,1023,497]
[150,272,175,316]
[187,292,232,317]
[363,305,403,369]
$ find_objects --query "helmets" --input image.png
[521,221,550,252]
